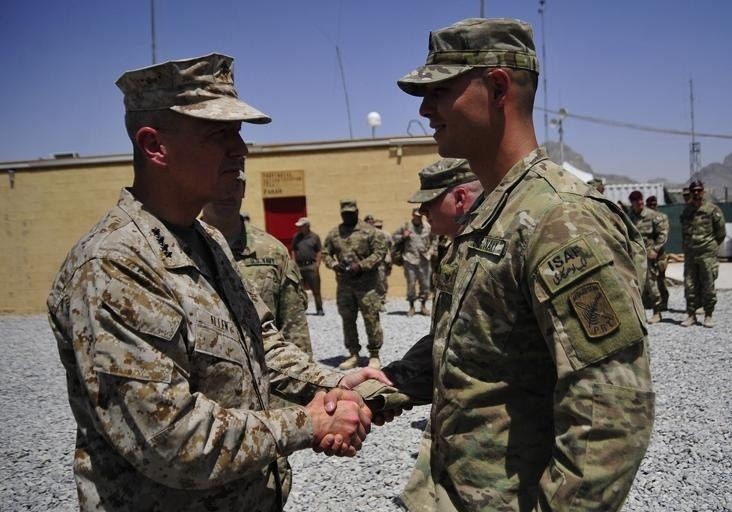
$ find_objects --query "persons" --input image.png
[314,17,654,512]
[392,208,434,317]
[682,187,693,204]
[200,158,314,363]
[587,177,605,195]
[320,198,387,369]
[680,181,726,328]
[374,220,393,312]
[628,191,667,323]
[407,158,484,235]
[290,218,326,315]
[46,51,371,512]
[364,214,374,225]
[646,196,669,312]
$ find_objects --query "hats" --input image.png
[683,187,691,195]
[116,53,272,125]
[295,218,310,226]
[408,158,479,203]
[413,208,423,216]
[689,181,704,191]
[237,157,245,181]
[398,17,539,96]
[340,199,356,212]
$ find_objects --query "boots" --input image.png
[681,313,697,326]
[338,354,360,369]
[407,306,415,316]
[648,310,662,323]
[421,303,430,316]
[368,351,380,368]
[704,310,714,329]
